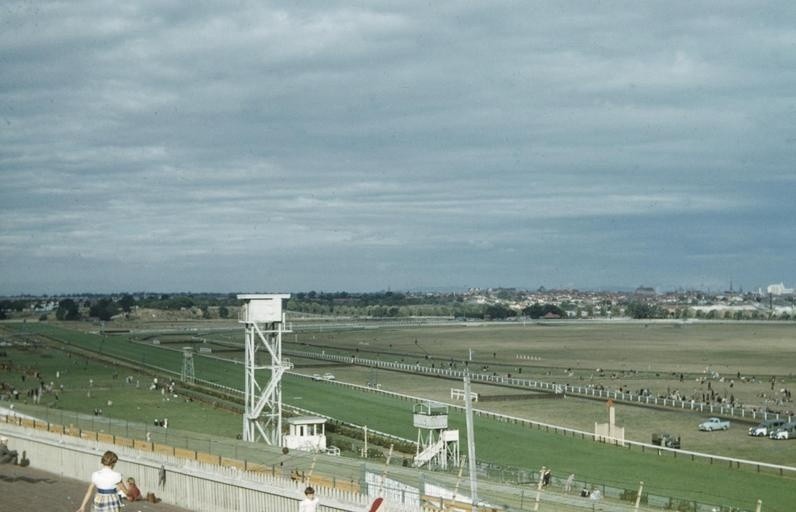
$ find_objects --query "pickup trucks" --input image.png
[698,417,731,432]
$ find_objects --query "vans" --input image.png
[746,418,786,436]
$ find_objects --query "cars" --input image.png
[323,372,336,380]
[312,373,323,381]
[767,420,796,439]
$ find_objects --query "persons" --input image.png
[299,487,320,512]
[542,469,551,487]
[0,436,18,465]
[564,474,575,492]
[77,450,131,512]
[126,477,141,502]
[158,465,166,490]
